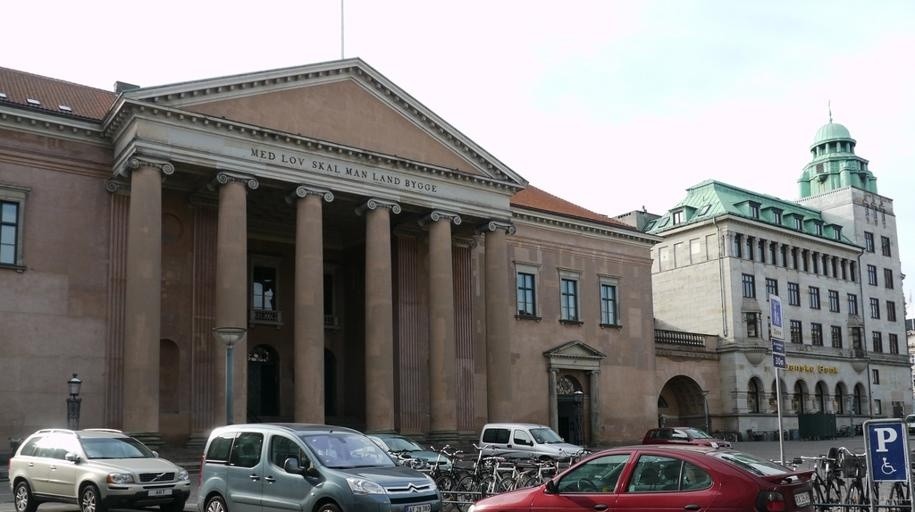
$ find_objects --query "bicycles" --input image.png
[801,446,915,512]
[386,444,596,512]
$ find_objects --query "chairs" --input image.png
[237,442,257,467]
[638,461,709,491]
[277,438,299,468]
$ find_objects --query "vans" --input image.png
[197,422,443,512]
[905,414,915,434]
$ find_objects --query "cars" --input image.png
[466,442,818,512]
[642,426,733,450]
[367,433,452,471]
[8,428,191,512]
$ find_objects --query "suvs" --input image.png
[478,422,586,476]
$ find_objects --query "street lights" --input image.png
[66,373,82,430]
[574,385,584,445]
[213,327,247,425]
